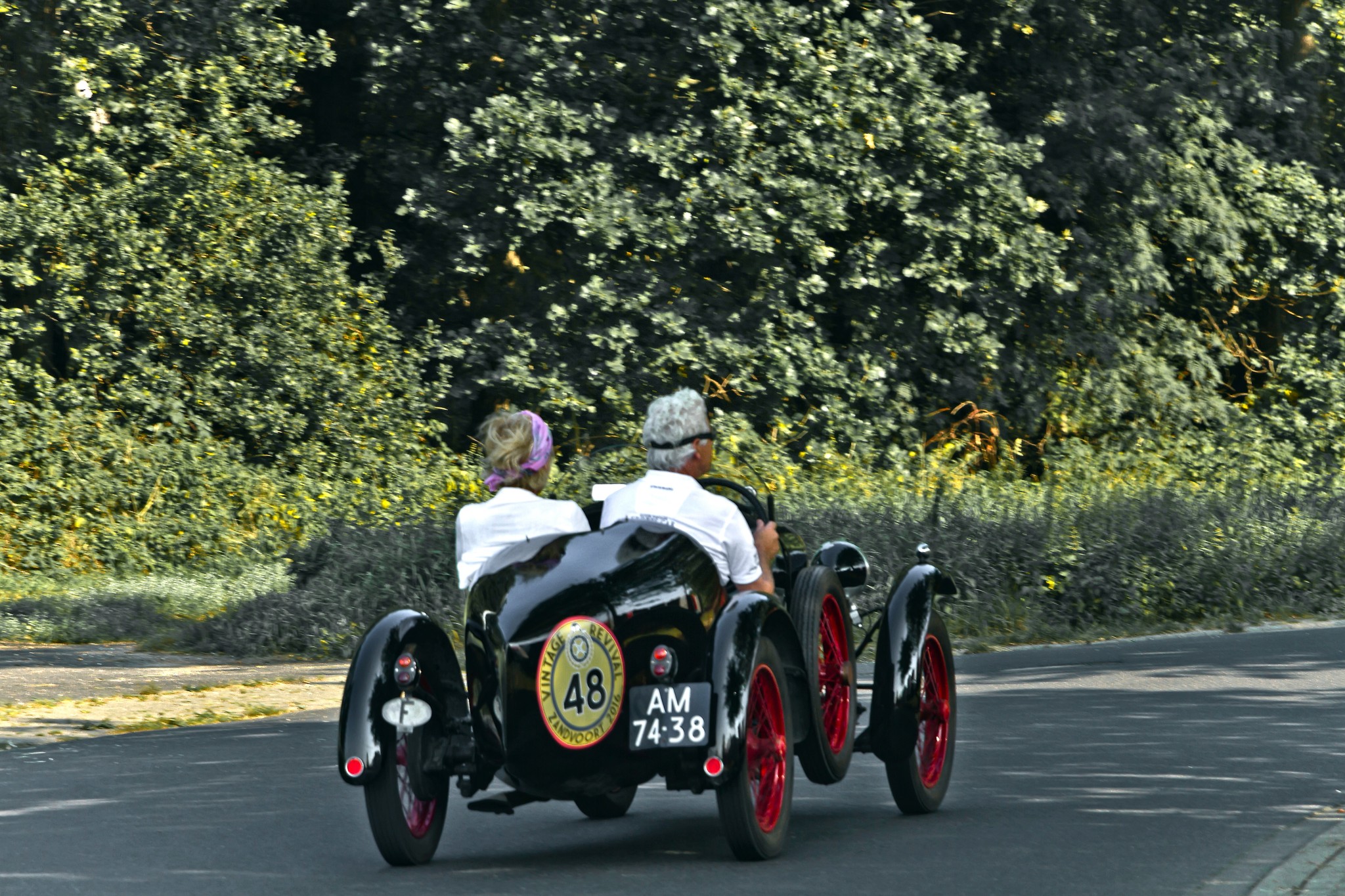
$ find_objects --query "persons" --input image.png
[598,385,782,596]
[453,408,592,594]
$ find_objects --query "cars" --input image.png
[336,476,960,870]
